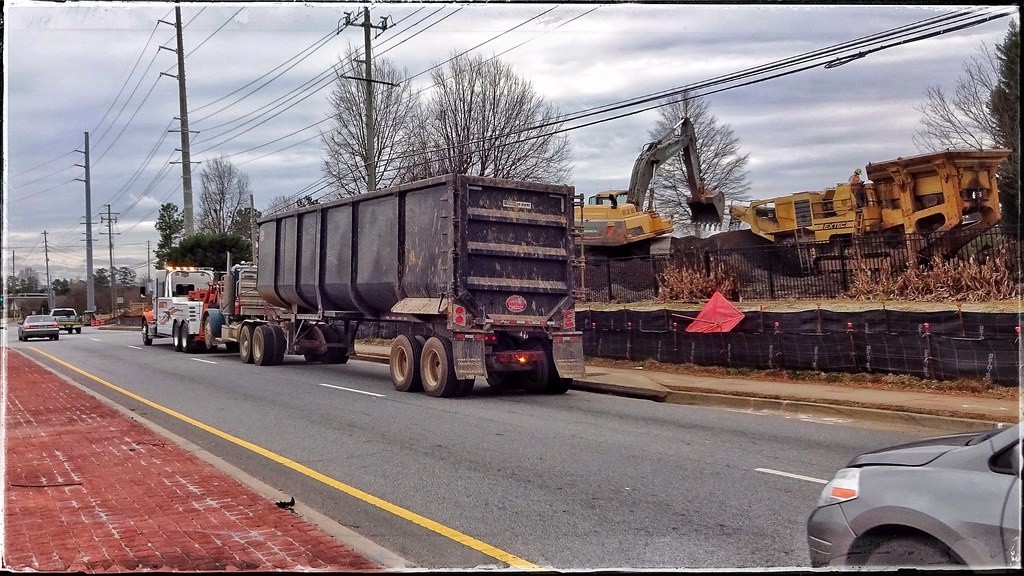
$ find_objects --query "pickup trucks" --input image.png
[48,308,82,334]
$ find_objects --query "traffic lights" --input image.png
[0,297,3,302]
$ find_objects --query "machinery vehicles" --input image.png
[574,116,726,296]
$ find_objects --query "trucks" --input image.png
[141,266,240,354]
[193,172,587,399]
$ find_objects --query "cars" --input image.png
[17,315,59,342]
[807,420,1021,568]
